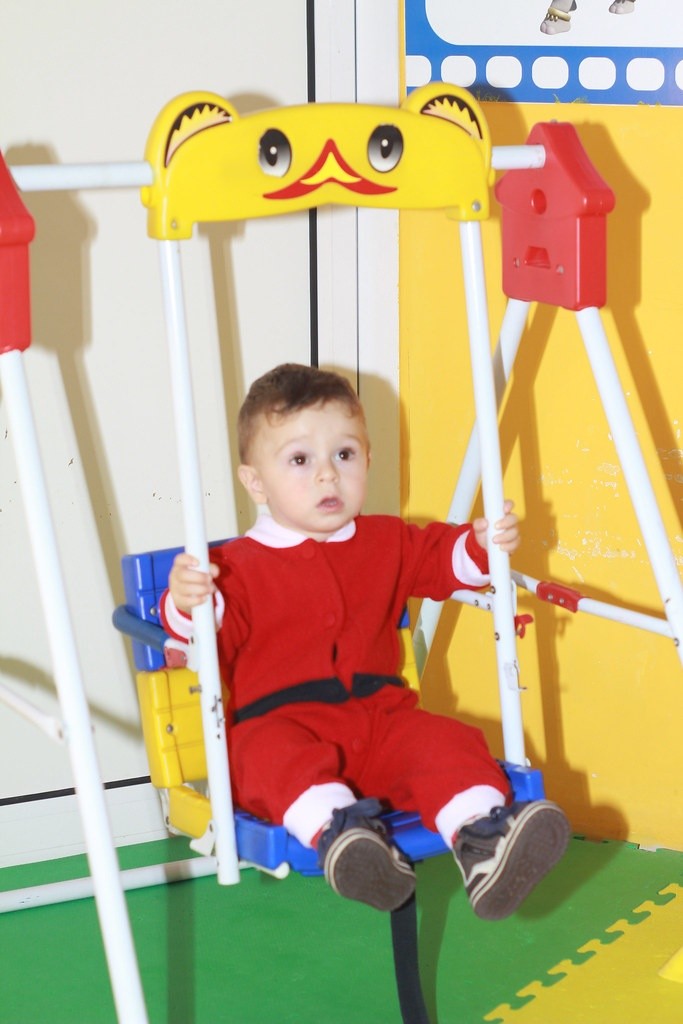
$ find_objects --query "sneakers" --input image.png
[317,797,417,912]
[452,790,569,920]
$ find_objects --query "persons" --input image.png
[162,364,569,920]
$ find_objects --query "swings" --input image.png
[108,186,551,888]
